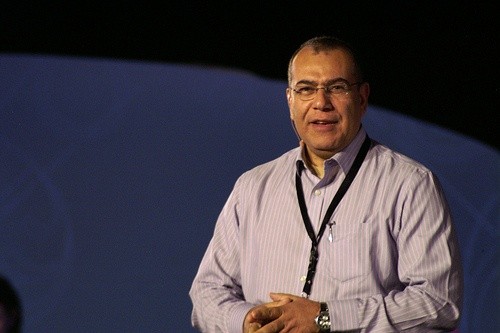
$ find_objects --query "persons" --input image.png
[188,34,465,333]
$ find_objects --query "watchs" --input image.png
[314,301,334,332]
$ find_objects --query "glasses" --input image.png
[288,79,362,100]
